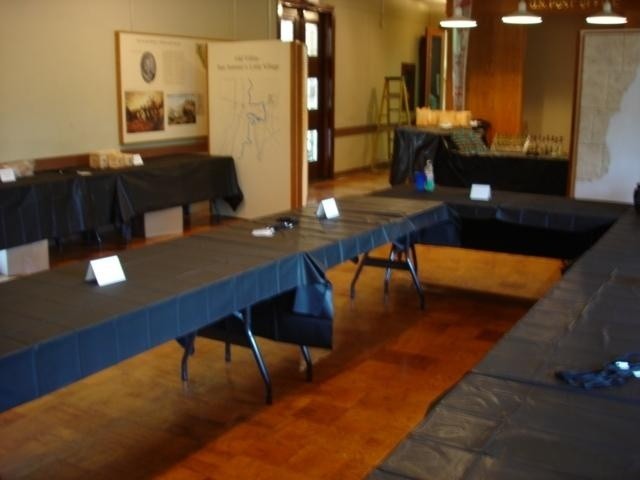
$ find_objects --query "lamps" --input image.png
[586,0,628,25]
[501,0,543,24]
[440,0,478,28]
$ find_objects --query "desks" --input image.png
[0,153,232,250]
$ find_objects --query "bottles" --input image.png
[423,159,435,192]
[492,131,567,156]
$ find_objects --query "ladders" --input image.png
[370,76,412,177]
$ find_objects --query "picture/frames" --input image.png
[114,31,240,146]
[567,28,640,203]
